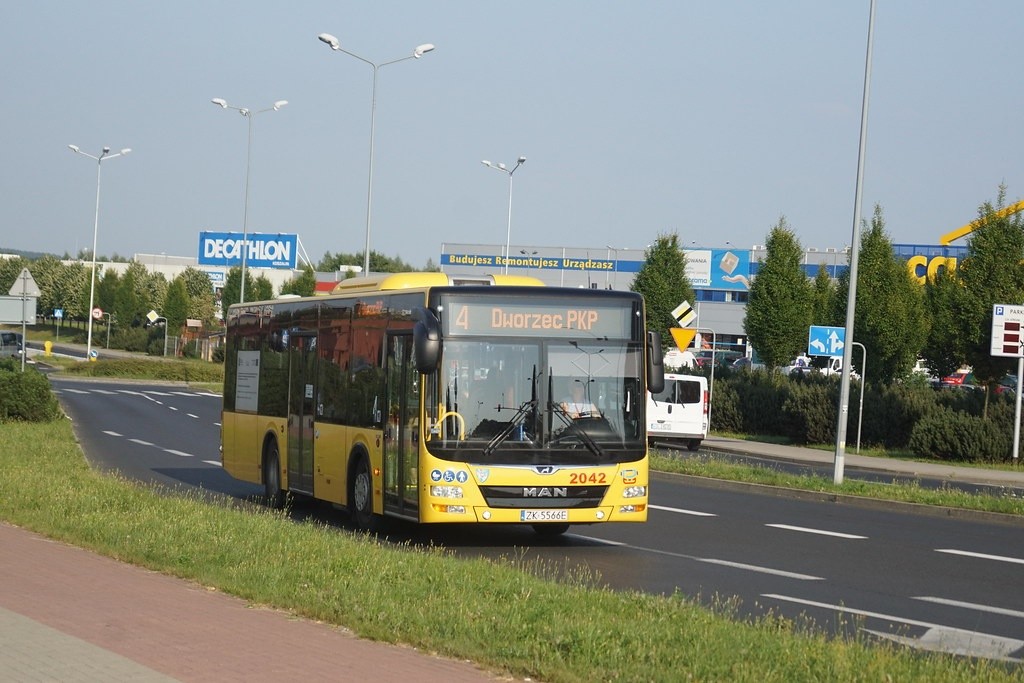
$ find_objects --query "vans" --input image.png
[598,372,708,451]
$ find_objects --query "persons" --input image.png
[555,378,602,429]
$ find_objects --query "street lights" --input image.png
[318,33,435,277]
[481,156,527,274]
[68,142,133,361]
[210,97,288,303]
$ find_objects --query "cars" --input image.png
[693,348,1024,404]
[0,329,26,364]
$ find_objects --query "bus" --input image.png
[218,271,665,540]
